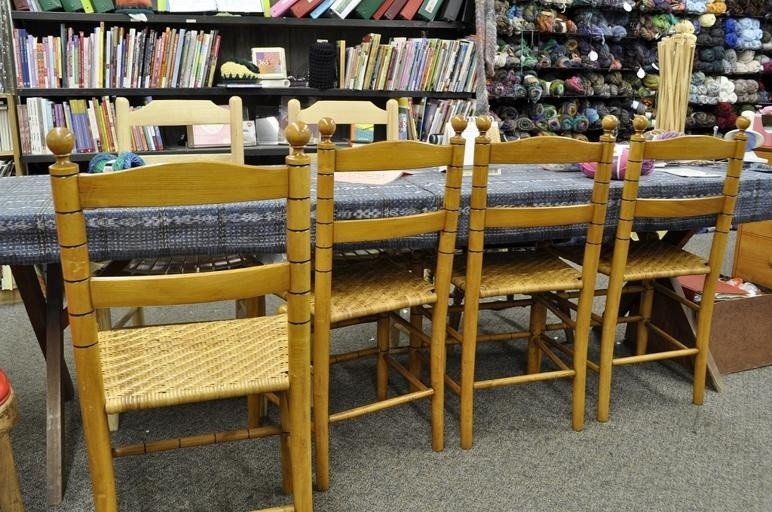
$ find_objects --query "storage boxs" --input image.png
[624,272,772,375]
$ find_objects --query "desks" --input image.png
[2,158,772,511]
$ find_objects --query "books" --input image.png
[257,106,279,145]
[1,97,165,153]
[12,21,221,90]
[672,273,750,306]
[334,32,479,93]
[187,105,256,147]
[278,95,321,145]
[398,97,478,146]
